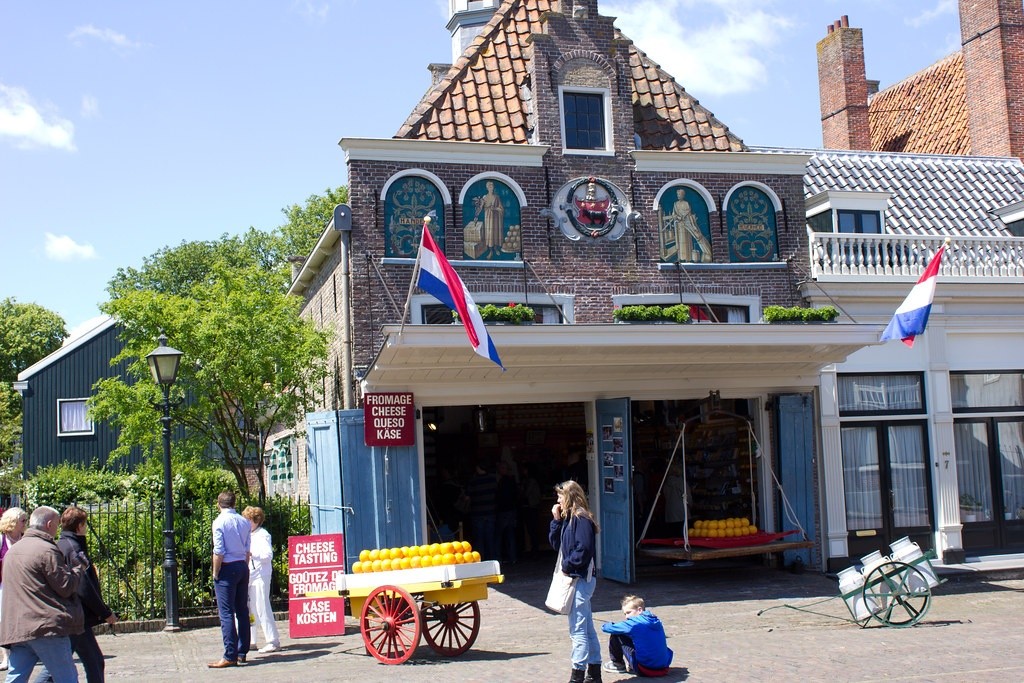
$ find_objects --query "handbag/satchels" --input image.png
[544,573,577,615]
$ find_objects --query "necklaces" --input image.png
[6,533,21,543]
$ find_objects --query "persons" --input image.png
[601,595,673,678]
[242,506,281,653]
[439,447,693,568]
[549,480,603,683]
[207,492,251,669]
[0,505,117,683]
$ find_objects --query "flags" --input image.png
[879,246,945,348]
[415,223,507,371]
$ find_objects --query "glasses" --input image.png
[21,519,28,522]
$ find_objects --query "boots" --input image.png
[568,669,585,683]
[583,664,603,683]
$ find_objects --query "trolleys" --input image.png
[754,534,948,631]
[334,542,504,667]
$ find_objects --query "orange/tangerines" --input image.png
[352,541,481,572]
[688,519,757,536]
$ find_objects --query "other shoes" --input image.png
[207,658,238,668]
[238,657,246,663]
[258,642,281,653]
[603,661,627,673]
[249,643,257,650]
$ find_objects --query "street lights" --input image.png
[145,333,186,633]
